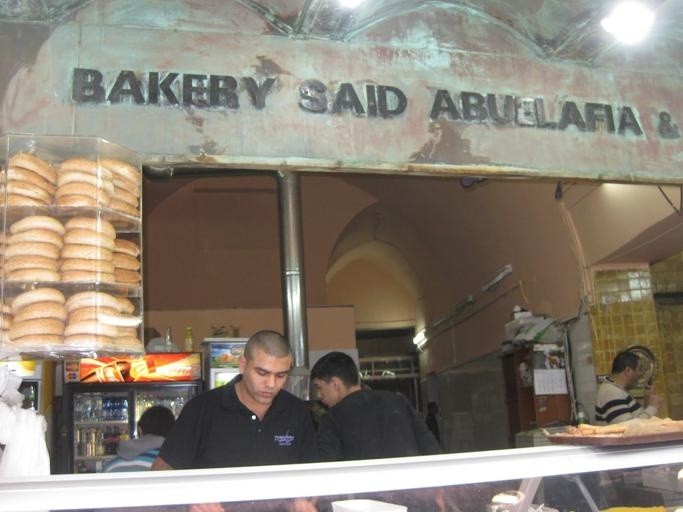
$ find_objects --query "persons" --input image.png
[151,329,319,511]
[97,403,178,472]
[593,349,664,424]
[309,352,463,512]
[422,400,443,442]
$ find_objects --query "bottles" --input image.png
[164,326,174,352]
[27,384,37,408]
[184,326,195,353]
[79,355,157,384]
[576,411,588,426]
[104,425,120,455]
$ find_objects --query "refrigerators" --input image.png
[52,349,206,474]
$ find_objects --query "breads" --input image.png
[0,154,142,346]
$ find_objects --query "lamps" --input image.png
[413,265,514,345]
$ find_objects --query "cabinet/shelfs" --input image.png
[498,341,575,438]
[0,133,143,361]
